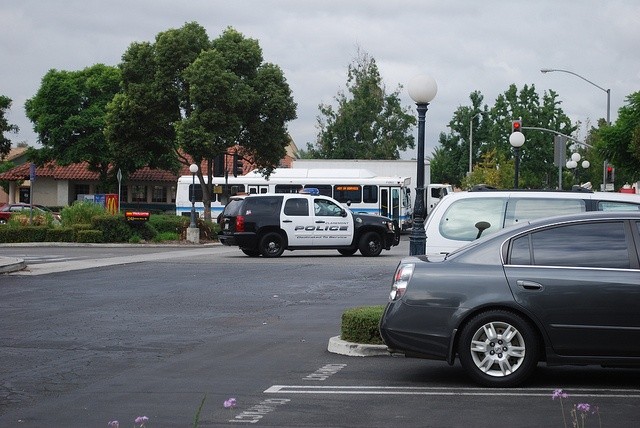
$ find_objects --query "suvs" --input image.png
[217,188,401,258]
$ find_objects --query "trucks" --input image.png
[292,158,455,216]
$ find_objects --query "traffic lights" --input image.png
[510,119,522,131]
[607,166,615,183]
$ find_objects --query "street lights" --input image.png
[408,72,438,256]
[509,131,525,187]
[29,163,36,219]
[187,163,200,244]
[541,69,610,124]
[469,111,492,172]
[565,153,590,184]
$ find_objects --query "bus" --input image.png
[175,175,413,234]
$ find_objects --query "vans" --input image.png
[422,190,640,255]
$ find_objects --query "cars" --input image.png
[0,203,62,224]
[379,210,640,388]
